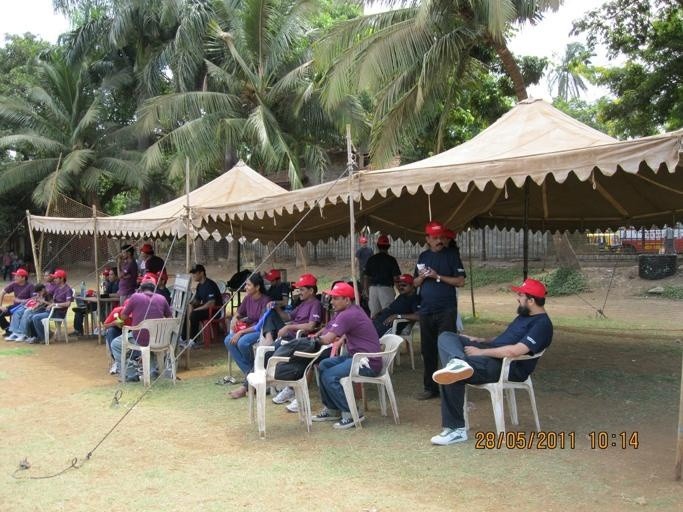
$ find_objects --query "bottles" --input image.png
[79,280,86,297]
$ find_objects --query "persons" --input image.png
[2,249,35,282]
[223,270,382,431]
[430,279,554,447]
[1,268,74,344]
[663,227,677,255]
[68,244,223,381]
[353,230,464,339]
[412,222,466,401]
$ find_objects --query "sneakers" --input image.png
[272,385,365,429]
[415,391,439,400]
[4,332,38,344]
[68,330,83,337]
[432,358,474,385]
[431,427,468,446]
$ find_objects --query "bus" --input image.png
[584,224,682,255]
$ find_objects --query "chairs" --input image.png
[247,342,326,440]
[464,343,547,441]
[121,318,180,386]
[193,293,229,345]
[334,333,404,428]
[391,318,414,370]
[41,287,76,344]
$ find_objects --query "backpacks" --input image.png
[229,269,251,289]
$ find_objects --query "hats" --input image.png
[359,236,389,246]
[512,279,548,298]
[49,270,67,280]
[11,269,28,278]
[323,283,354,300]
[292,275,317,287]
[189,264,204,273]
[32,284,45,293]
[425,221,454,238]
[394,274,412,284]
[142,270,168,285]
[265,270,281,281]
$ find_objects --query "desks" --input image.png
[75,295,121,344]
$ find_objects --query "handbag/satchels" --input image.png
[272,336,322,380]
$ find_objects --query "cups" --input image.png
[416,264,425,272]
